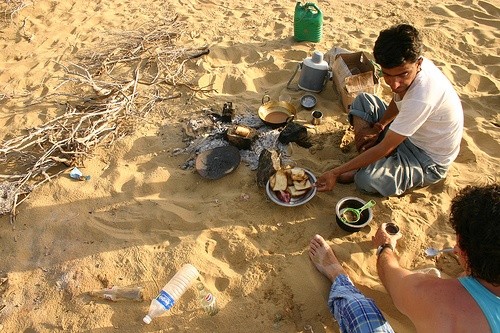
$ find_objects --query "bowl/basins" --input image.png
[300,94,317,110]
[335,196,374,233]
[266,167,317,207]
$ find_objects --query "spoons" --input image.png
[424,247,454,256]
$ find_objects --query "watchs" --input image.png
[376,244,393,256]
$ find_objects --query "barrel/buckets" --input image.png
[297,51,330,93]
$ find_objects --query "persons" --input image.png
[309,24,464,199]
[307,183,500,333]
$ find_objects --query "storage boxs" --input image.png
[332,51,380,113]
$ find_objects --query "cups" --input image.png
[311,110,323,125]
[385,222,399,235]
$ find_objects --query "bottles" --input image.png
[89,286,144,302]
[201,293,218,316]
[294,2,323,42]
[143,263,200,325]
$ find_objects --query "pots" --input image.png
[258,95,297,126]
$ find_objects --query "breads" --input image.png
[269,168,312,198]
[234,127,250,138]
